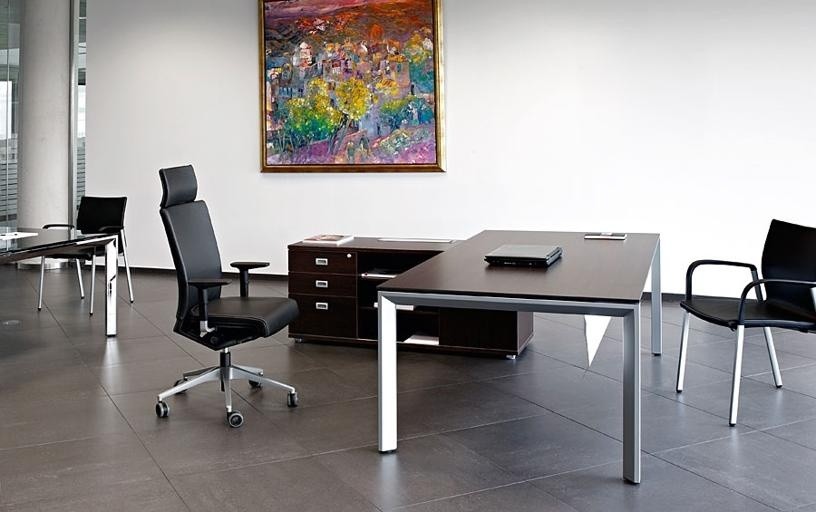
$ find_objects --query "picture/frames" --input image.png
[257,0,445,174]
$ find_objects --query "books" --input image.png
[0,230,38,241]
[404,332,439,346]
[373,299,417,312]
[302,232,355,249]
[361,266,401,281]
[585,230,629,241]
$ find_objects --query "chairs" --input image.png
[153,165,301,427]
[676,219,816,426]
[30,197,135,314]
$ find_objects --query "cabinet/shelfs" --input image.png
[355,247,441,352]
[438,307,533,355]
[288,246,357,345]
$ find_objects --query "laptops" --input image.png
[482,245,563,268]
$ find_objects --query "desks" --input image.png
[376,229,663,484]
[0,224,119,337]
